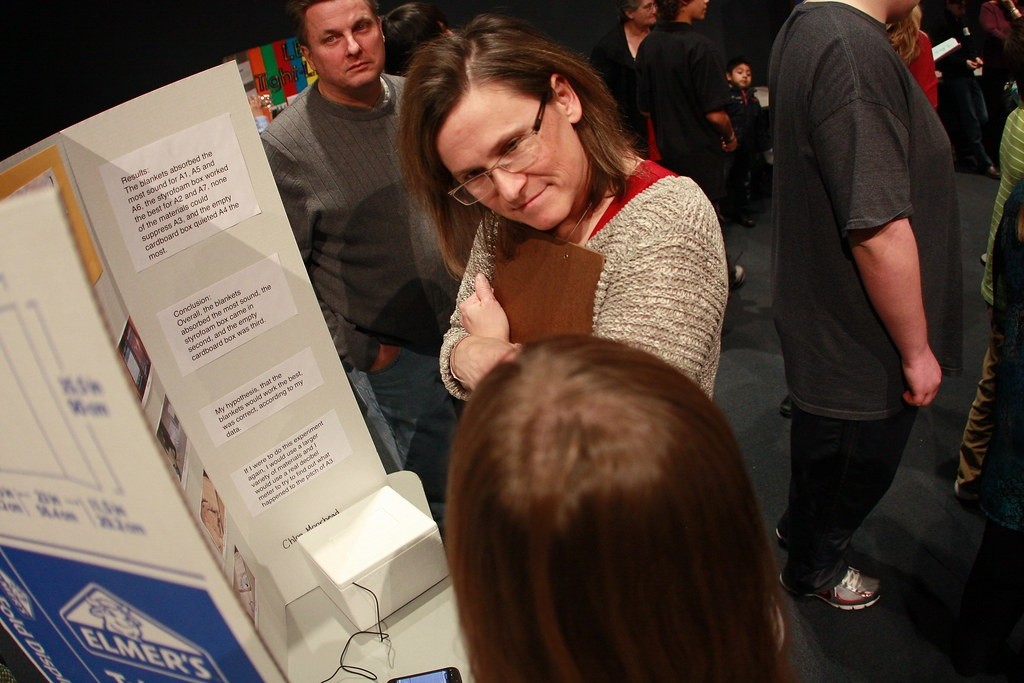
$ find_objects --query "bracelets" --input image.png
[1009,7,1019,16]
[722,133,735,144]
[450,334,471,383]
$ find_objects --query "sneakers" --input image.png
[775,526,792,549]
[779,564,882,611]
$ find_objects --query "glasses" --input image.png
[447,87,548,208]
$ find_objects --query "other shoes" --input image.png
[980,252,987,264]
[985,163,1001,180]
[779,394,794,416]
[953,480,978,500]
[728,264,746,298]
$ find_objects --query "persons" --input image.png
[445,334,825,683]
[954,43,1024,683]
[588,0,1024,417]
[259,0,467,543]
[401,15,729,402]
[768,1,963,609]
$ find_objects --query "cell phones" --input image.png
[932,37,963,61]
[387,667,462,683]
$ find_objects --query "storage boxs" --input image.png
[296,485,449,632]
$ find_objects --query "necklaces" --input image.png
[566,201,594,240]
